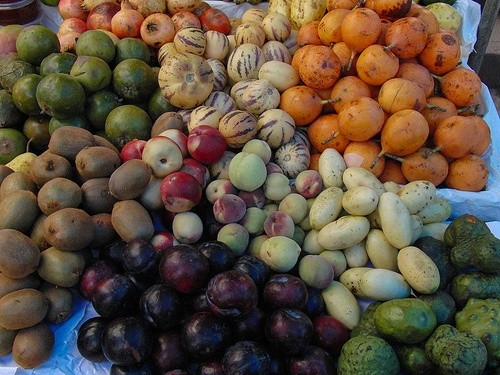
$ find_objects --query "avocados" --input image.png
[337,211,500,375]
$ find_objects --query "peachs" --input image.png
[118,125,347,291]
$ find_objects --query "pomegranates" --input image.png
[56,0,231,51]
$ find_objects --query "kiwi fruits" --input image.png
[0,126,155,370]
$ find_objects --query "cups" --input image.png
[0,0,40,27]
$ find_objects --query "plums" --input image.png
[78,238,350,375]
